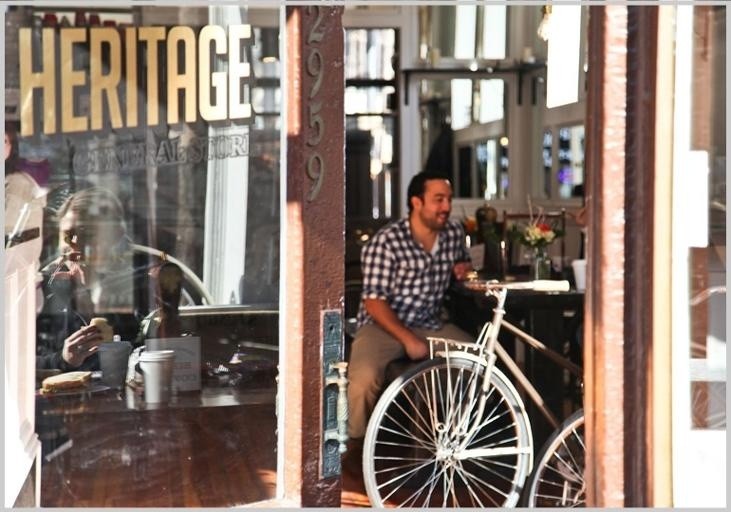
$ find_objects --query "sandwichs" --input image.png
[39,370,93,395]
[81,317,112,342]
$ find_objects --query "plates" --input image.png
[36,384,111,396]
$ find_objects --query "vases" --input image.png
[530,248,550,283]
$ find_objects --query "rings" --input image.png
[77,344,85,352]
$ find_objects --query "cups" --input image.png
[97,341,132,390]
[571,259,587,290]
[138,350,177,404]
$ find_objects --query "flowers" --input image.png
[511,200,562,249]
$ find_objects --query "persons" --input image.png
[344,168,480,473]
[32,189,191,508]
[3,121,73,466]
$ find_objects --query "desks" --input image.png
[452,280,591,469]
